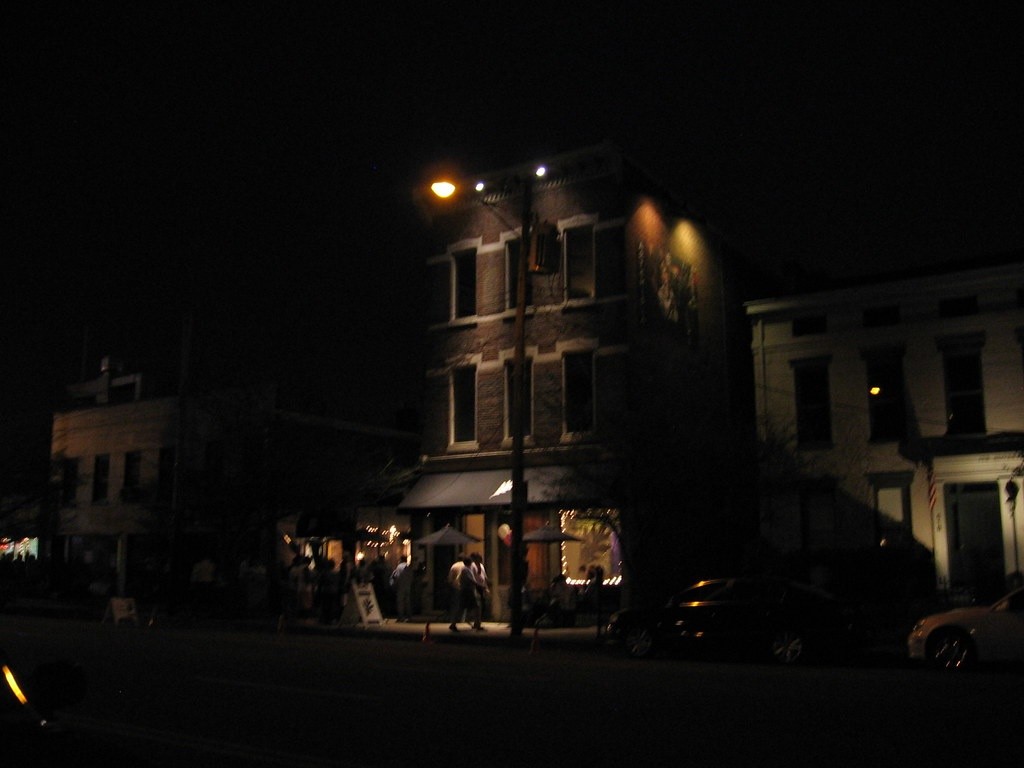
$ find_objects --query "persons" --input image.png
[275,554,606,639]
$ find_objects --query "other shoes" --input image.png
[449,625,458,632]
[474,623,483,630]
[406,620,412,623]
[396,619,404,622]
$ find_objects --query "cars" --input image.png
[906,585,1024,674]
[602,574,856,669]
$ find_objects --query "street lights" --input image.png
[424,151,548,635]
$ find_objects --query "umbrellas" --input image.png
[522,521,584,587]
[413,523,481,573]
[399,530,411,544]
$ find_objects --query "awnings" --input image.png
[346,529,389,544]
[395,464,626,516]
